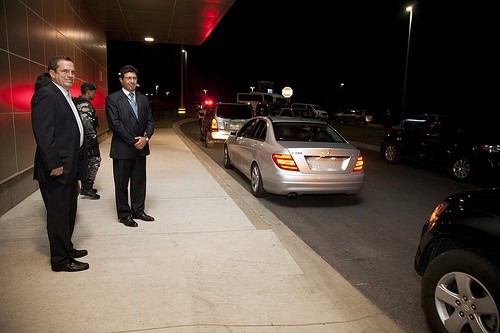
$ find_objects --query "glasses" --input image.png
[56,69,77,75]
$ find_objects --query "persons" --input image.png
[71,82,102,200]
[31,55,89,272]
[104,65,154,227]
[35,73,51,92]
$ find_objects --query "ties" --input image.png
[129,93,138,119]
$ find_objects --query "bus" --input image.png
[236,93,287,113]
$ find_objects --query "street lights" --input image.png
[402,5,412,106]
[181,49,187,109]
[250,87,255,92]
[203,89,209,98]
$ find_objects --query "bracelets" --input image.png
[144,136,149,142]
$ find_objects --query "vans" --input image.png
[291,103,328,119]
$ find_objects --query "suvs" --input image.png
[255,101,284,117]
[332,108,374,124]
[200,103,255,148]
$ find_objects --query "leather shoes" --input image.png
[71,248,88,258]
[133,213,154,221]
[52,259,89,271]
[120,215,138,227]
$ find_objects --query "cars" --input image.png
[380,119,500,182]
[401,113,445,128]
[413,186,500,333]
[222,115,365,198]
[275,107,323,120]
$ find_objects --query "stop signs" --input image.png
[282,87,293,97]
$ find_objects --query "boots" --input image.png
[81,179,100,199]
